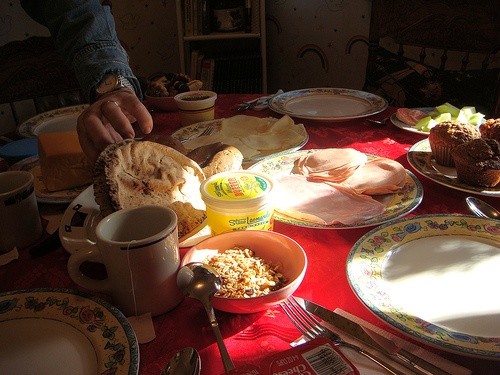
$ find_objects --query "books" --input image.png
[190,52,214,91]
[184,0,209,36]
[246,0,259,33]
[214,54,261,94]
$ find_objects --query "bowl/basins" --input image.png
[181,229,308,314]
[144,88,176,110]
[0,138,37,165]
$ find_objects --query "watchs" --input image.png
[90,73,135,105]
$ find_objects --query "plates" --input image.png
[20,105,93,140]
[247,147,423,231]
[390,107,487,135]
[7,154,92,205]
[345,212,499,363]
[0,288,140,374]
[166,117,311,165]
[268,87,388,122]
[57,183,212,245]
[405,137,500,199]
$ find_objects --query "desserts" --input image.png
[479,118,500,143]
[429,121,481,167]
[451,138,500,187]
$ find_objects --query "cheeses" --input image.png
[38,131,92,191]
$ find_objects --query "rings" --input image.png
[102,100,117,111]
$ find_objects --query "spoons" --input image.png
[466,197,500,220]
[162,346,202,375]
[366,116,390,126]
[176,262,239,375]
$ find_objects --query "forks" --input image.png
[280,295,407,375]
[197,127,214,137]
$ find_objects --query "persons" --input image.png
[21,0,153,161]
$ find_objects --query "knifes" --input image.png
[291,296,452,375]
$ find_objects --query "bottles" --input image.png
[199,170,276,234]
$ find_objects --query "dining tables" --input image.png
[0,94,500,375]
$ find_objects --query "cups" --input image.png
[67,205,182,316]
[0,171,42,254]
[174,90,217,129]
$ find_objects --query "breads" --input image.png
[185,143,244,179]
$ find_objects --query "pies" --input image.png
[93,139,208,239]
